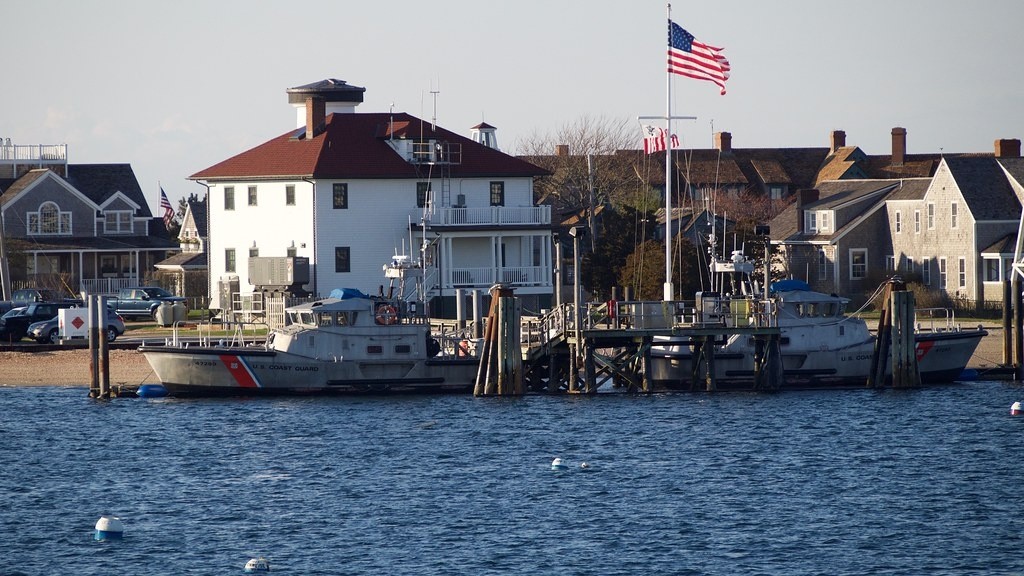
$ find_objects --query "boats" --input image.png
[132,154,484,396]
[613,2,991,386]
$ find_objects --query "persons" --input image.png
[376,285,384,297]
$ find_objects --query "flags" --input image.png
[667,19,731,96]
[159,187,174,226]
[640,125,679,154]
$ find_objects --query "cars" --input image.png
[26,304,126,345]
[0,287,114,342]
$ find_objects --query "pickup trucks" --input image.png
[99,286,190,323]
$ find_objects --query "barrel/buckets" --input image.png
[173,301,187,325]
[730,299,750,326]
[457,338,483,356]
[157,301,173,325]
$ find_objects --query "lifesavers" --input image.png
[377,305,396,324]
